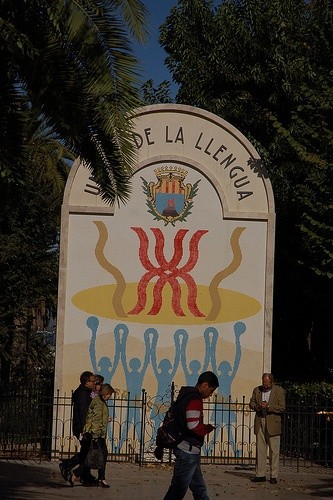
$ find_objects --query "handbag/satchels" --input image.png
[84,437,106,470]
[156,418,186,449]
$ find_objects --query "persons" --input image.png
[57,371,114,488]
[160,371,220,500]
[248,372,285,484]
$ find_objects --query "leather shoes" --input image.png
[270,478,277,484]
[251,476,266,482]
[59,462,69,480]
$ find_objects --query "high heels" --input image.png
[96,477,111,488]
[68,475,79,486]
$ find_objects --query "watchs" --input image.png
[266,405,269,411]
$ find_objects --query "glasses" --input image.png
[87,380,96,384]
[262,383,271,388]
